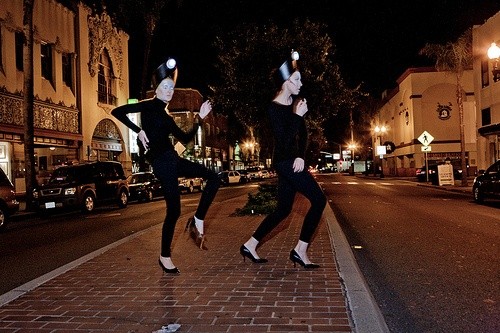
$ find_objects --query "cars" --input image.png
[177,166,278,194]
[307,159,383,177]
[472,159,500,204]
[126,172,164,203]
[0,167,19,234]
[415,159,470,186]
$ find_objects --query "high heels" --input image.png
[159,258,180,273]
[186,215,206,246]
[290,249,320,268]
[240,244,268,263]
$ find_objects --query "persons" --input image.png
[110,59,222,276]
[240,47,328,268]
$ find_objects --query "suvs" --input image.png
[30,160,131,219]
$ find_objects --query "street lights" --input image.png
[375,125,386,178]
[325,140,342,160]
